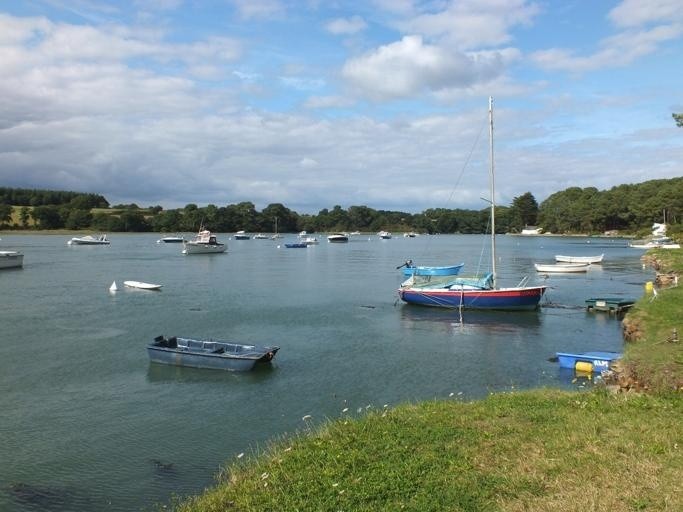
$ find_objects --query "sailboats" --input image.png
[401,94,550,310]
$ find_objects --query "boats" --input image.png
[555,254,604,264]
[397,259,464,275]
[124,280,162,290]
[146,333,279,372]
[629,242,681,249]
[533,262,590,273]
